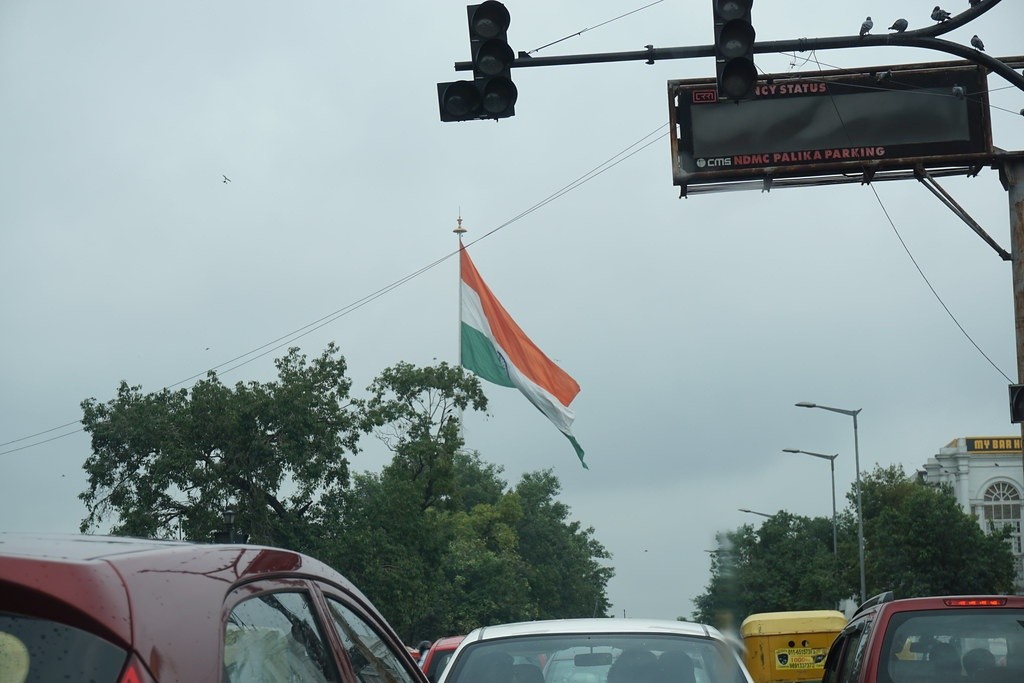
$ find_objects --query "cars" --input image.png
[0,532,430,683]
[542,646,622,683]
[438,618,755,683]
[421,635,547,683]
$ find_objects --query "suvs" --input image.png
[822,592,1024,683]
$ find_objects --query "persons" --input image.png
[929,643,959,662]
[658,651,696,683]
[962,648,995,674]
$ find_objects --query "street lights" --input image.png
[782,449,839,554]
[794,402,866,605]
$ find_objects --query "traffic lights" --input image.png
[437,1,518,123]
[713,0,758,99]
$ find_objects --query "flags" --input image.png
[460,240,590,471]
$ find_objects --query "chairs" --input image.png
[608,649,697,683]
[462,654,544,683]
[891,659,939,683]
